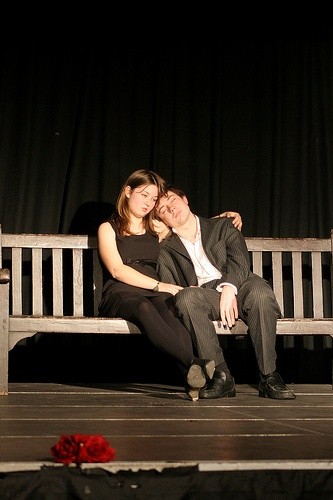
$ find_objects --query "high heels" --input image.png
[259,372,296,399]
[192,358,215,379]
[200,370,236,398]
[186,364,206,401]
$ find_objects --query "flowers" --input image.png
[48,434,115,469]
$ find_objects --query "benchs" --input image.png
[0,234,333,396]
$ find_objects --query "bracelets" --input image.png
[152,281,161,293]
[218,213,221,218]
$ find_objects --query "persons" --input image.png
[153,188,296,402]
[96,170,244,398]
